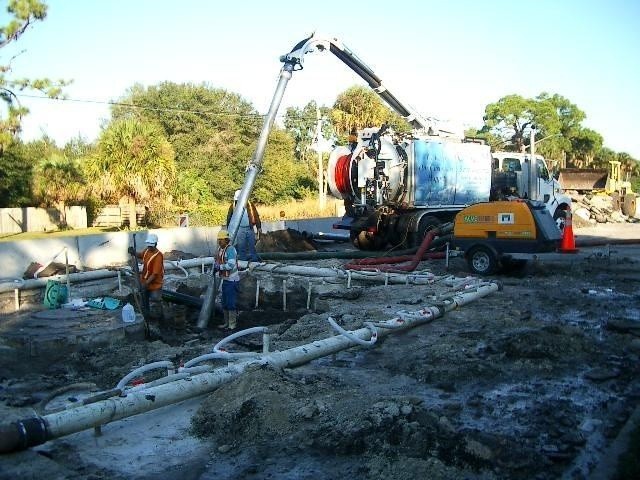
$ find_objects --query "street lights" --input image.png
[518,133,563,153]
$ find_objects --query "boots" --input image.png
[218,309,236,329]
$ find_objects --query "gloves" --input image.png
[214,263,220,269]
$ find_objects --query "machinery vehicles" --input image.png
[327,122,572,255]
[556,161,637,218]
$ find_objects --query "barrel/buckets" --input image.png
[121,302,135,322]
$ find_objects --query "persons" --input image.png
[226,188,264,263]
[212,230,240,331]
[128,233,164,341]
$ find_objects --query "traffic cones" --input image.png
[559,205,580,252]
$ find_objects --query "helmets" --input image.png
[216,230,230,239]
[234,190,242,199]
[144,235,159,247]
